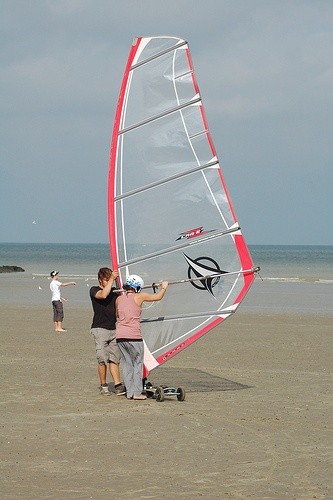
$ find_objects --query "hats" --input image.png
[51,271,59,277]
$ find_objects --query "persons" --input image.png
[115,274,169,400]
[89,267,128,397]
[49,271,77,332]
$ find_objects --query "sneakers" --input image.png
[114,384,127,395]
[99,385,112,396]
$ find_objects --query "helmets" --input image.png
[123,274,144,294]
